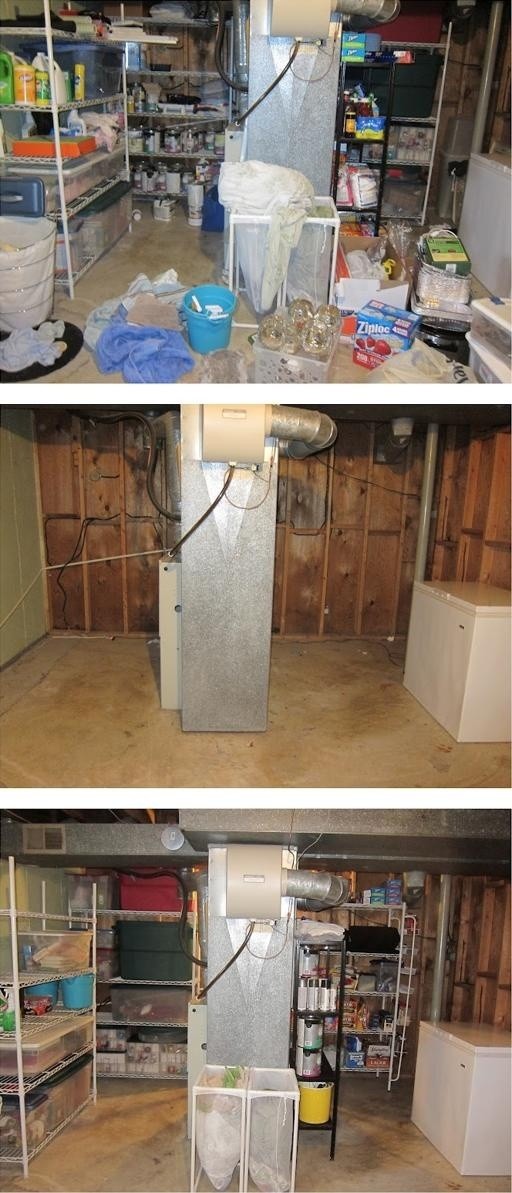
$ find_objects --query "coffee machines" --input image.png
[410,1018,512,1177]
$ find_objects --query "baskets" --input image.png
[247,320,345,383]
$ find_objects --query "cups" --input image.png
[204,348,249,383]
[256,296,342,356]
[132,208,142,220]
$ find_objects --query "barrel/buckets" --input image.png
[31,51,68,108]
[61,973,95,1009]
[299,1082,334,1125]
[186,184,204,227]
[0,47,15,104]
[182,282,238,354]
[25,981,59,1008]
[7,51,35,105]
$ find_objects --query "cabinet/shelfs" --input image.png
[0,0,234,300]
[332,21,452,237]
[289,902,421,1161]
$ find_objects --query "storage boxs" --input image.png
[470,297,512,371]
[0,856,201,1179]
[465,331,512,384]
[330,235,413,344]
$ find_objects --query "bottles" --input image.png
[344,90,374,138]
[115,100,124,130]
[134,162,167,190]
[302,948,311,977]
[20,110,38,138]
[128,85,158,112]
[379,995,390,1028]
[75,62,86,101]
[396,129,430,162]
[298,973,337,1010]
[133,127,226,153]
[65,108,88,136]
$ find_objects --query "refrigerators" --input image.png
[399,578,512,743]
[456,150,512,301]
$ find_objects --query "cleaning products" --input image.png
[67,109,85,135]
[21,111,38,137]
